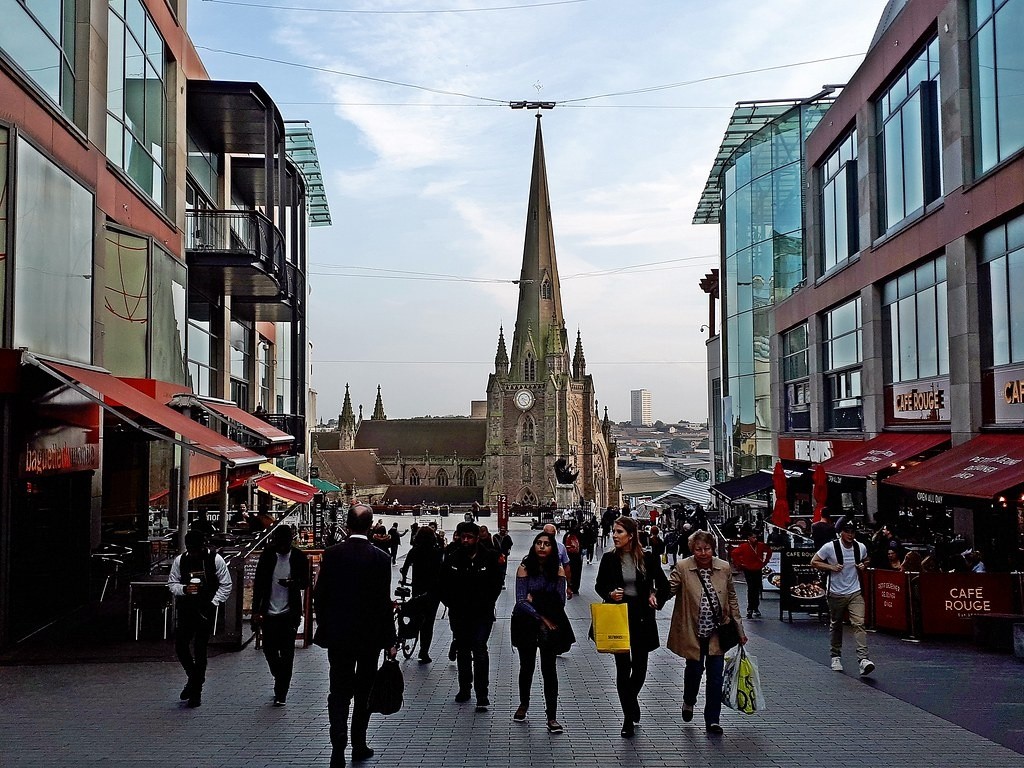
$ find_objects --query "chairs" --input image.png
[89,513,287,641]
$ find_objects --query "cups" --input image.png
[190,578,201,594]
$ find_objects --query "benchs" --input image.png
[370,504,431,515]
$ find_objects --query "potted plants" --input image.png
[413,505,423,516]
[468,506,490,515]
[431,508,440,515]
[440,505,449,517]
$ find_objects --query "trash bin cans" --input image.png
[413,506,420,515]
[440,506,448,516]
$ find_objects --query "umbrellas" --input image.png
[772,458,791,529]
[811,462,829,524]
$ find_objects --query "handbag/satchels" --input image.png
[362,655,405,715]
[662,545,668,564]
[250,605,269,625]
[720,641,768,716]
[716,620,740,653]
[590,592,631,653]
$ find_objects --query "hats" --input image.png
[840,518,857,530]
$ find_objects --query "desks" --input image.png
[905,545,928,557]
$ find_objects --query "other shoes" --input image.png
[706,723,723,736]
[455,690,472,703]
[418,651,433,663]
[682,702,693,723]
[631,697,641,723]
[754,609,761,616]
[621,719,634,738]
[351,745,374,763]
[476,696,489,709]
[329,749,346,768]
[747,612,752,618]
[274,697,287,707]
[180,678,205,708]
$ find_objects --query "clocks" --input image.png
[513,388,535,412]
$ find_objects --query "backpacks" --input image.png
[565,532,580,553]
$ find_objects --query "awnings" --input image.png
[707,472,775,504]
[22,353,267,470]
[251,460,342,505]
[808,432,953,477]
[881,435,1024,500]
[194,395,296,444]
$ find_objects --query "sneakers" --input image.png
[859,659,875,675]
[513,704,529,722]
[547,718,562,733]
[831,656,843,671]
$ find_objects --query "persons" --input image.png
[809,520,875,676]
[438,523,504,706]
[166,530,232,707]
[250,524,309,706]
[513,532,564,734]
[313,505,396,767]
[228,503,263,533]
[595,516,669,738]
[376,500,907,666]
[649,530,748,734]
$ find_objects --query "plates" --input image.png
[767,573,780,589]
[791,587,825,599]
